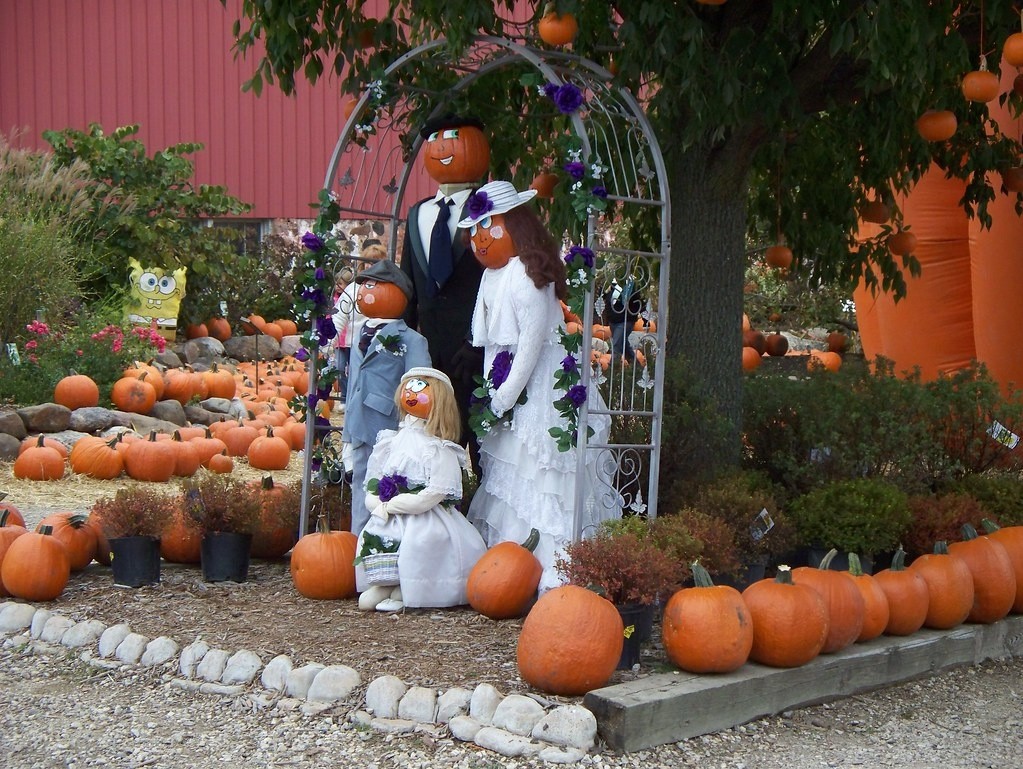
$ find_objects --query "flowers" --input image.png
[292,62,611,566]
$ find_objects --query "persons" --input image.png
[593,265,651,375]
[325,244,389,415]
[340,112,611,608]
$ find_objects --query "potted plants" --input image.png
[552,472,914,670]
[87,469,184,592]
[282,471,353,535]
[169,462,276,583]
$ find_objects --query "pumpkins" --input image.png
[0,308,360,600]
[342,10,655,420]
[467,520,1023,697]
[741,31,1021,374]
